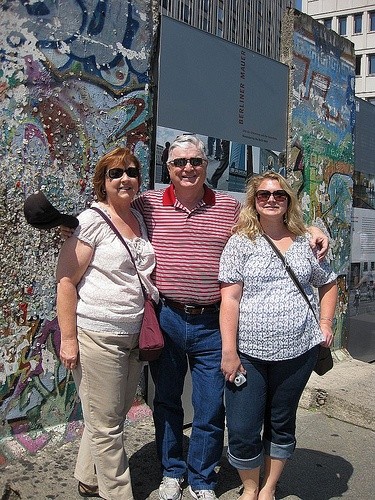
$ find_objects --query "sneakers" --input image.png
[189,484,218,500]
[159,476,184,500]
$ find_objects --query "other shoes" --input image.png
[78,481,101,497]
[237,489,275,500]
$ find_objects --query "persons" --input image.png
[218,171,338,500]
[59,134,329,500]
[56,147,161,500]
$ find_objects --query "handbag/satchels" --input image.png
[313,346,334,376]
[138,294,164,361]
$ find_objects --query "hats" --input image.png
[24,191,79,230]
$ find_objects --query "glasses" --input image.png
[168,158,209,167]
[254,190,289,201]
[105,167,139,179]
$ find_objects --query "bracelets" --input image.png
[320,316,335,328]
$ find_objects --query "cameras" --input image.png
[233,370,246,387]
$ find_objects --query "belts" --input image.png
[159,292,221,316]
